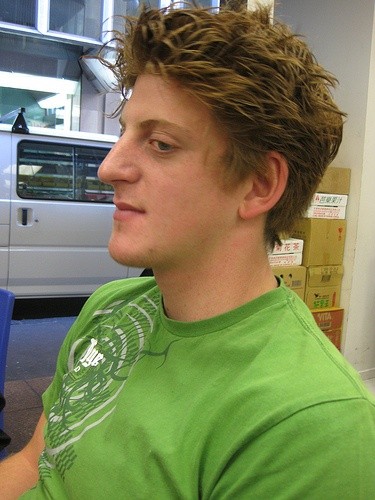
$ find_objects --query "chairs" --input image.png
[0,288,15,459]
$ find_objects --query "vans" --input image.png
[0,123,156,300]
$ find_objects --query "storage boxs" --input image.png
[288,205,346,266]
[304,167,350,205]
[306,266,343,309]
[271,267,306,302]
[264,237,304,266]
[307,308,344,331]
[319,330,342,354]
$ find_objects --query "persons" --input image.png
[0,1,375,499]
